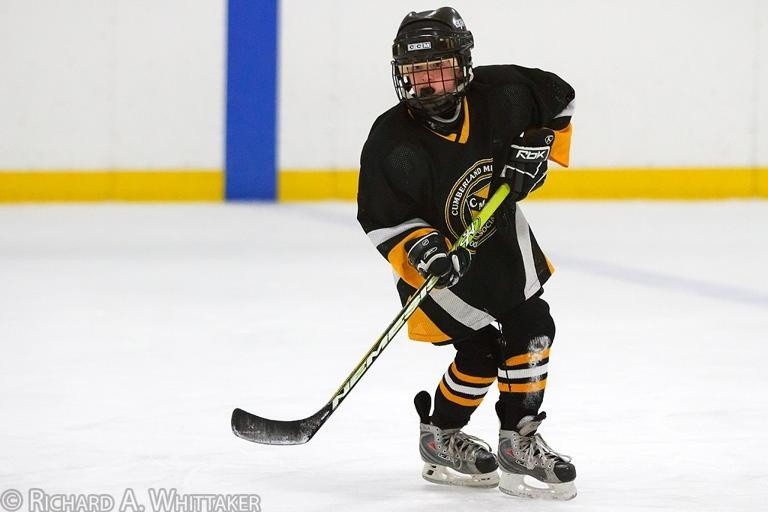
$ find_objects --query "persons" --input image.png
[357,7,576,483]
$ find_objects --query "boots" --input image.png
[497,411,576,483]
[414,391,498,474]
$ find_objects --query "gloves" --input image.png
[495,144,551,202]
[404,231,472,289]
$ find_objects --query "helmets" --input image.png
[391,7,474,66]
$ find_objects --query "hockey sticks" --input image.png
[231,183,510,445]
